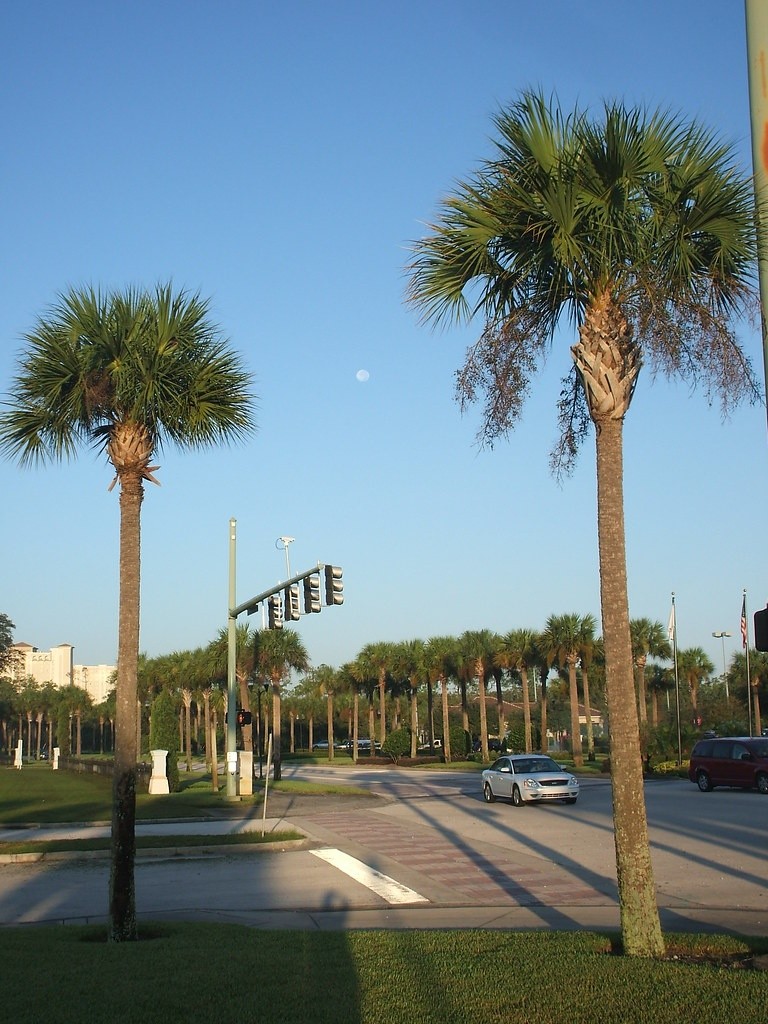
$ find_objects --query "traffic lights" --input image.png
[304,577,321,613]
[285,586,299,622]
[268,596,283,629]
[236,712,246,726]
[325,564,343,606]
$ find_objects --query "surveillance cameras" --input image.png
[281,537,295,542]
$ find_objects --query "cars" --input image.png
[313,740,337,750]
[424,740,442,748]
[481,754,580,807]
[366,743,381,749]
[359,742,371,750]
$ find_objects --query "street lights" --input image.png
[247,679,269,778]
[713,631,731,696]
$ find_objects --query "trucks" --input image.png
[338,739,375,750]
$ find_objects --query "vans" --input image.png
[688,737,768,794]
